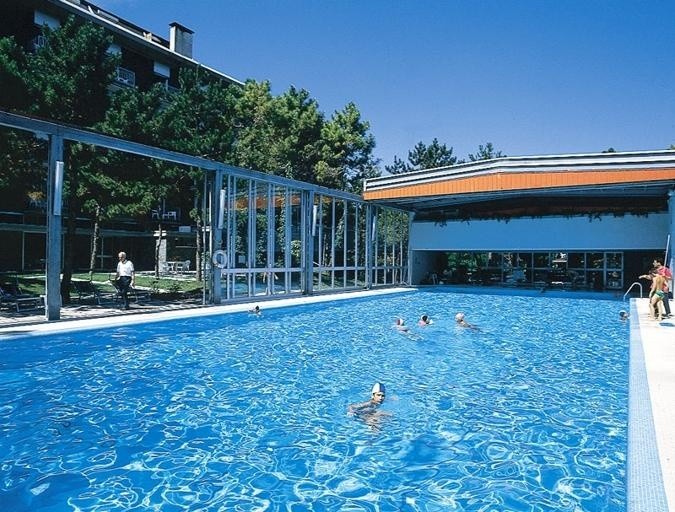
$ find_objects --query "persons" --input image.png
[593,272,604,290]
[61,248,76,304]
[391,318,424,343]
[347,382,393,431]
[115,252,136,310]
[417,314,430,325]
[263,263,279,294]
[649,267,669,321]
[456,313,480,330]
[249,305,261,317]
[618,309,633,322]
[665,265,673,299]
[638,256,673,317]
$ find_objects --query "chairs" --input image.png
[71,268,149,307]
[0,271,44,313]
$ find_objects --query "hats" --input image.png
[372,383,385,394]
[455,313,465,320]
[421,315,428,321]
[397,319,404,325]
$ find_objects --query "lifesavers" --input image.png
[214,250,229,269]
